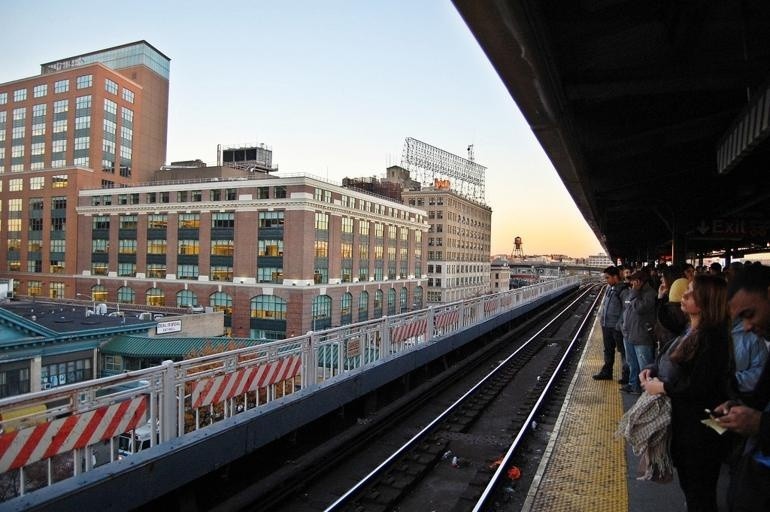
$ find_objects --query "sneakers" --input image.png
[593,372,613,380]
[619,385,641,395]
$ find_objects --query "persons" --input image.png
[591,260,770,512]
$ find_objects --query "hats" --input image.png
[626,271,649,282]
[668,278,689,303]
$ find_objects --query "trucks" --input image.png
[118,418,159,460]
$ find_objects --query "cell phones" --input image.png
[704,408,726,418]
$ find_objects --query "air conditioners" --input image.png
[42,384,51,390]
